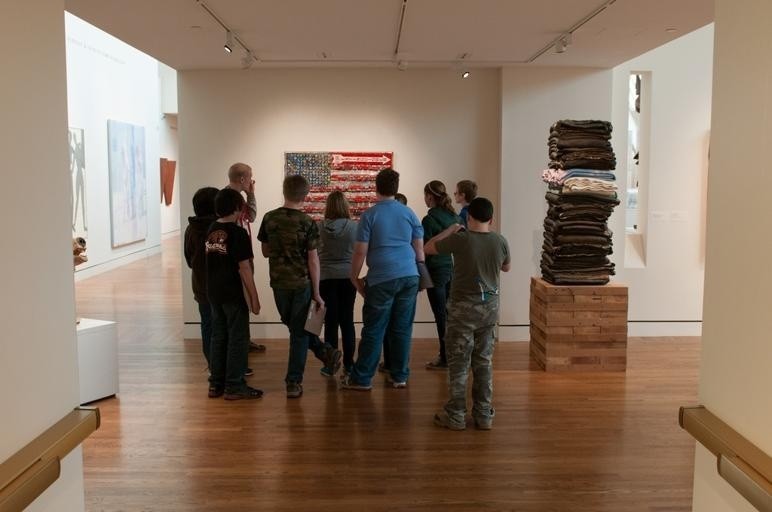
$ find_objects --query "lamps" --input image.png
[224,29,233,53]
[458,52,471,79]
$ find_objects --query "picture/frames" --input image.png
[107,119,149,249]
[69,126,88,237]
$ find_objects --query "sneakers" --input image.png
[284,376,304,399]
[207,376,224,398]
[248,341,266,353]
[223,377,264,401]
[471,407,495,430]
[424,354,447,370]
[319,366,332,377]
[378,356,409,373]
[339,373,372,390]
[244,367,251,374]
[343,364,353,376]
[323,346,342,376]
[432,410,467,431]
[384,370,408,388]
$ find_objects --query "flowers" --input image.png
[73,237,88,268]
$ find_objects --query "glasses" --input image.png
[454,192,458,195]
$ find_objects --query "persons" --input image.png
[378,193,407,372]
[222,163,266,352]
[424,197,511,431]
[340,168,424,390]
[184,187,253,376]
[317,190,359,376]
[454,180,478,223]
[258,176,342,398]
[421,180,465,368]
[205,190,264,401]
